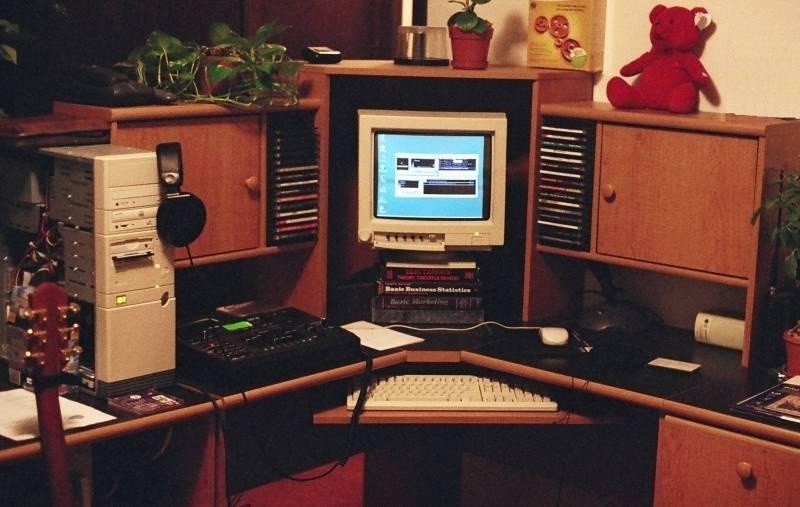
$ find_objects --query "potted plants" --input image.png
[114,20,302,109]
[447,0,493,69]
[749,166,800,376]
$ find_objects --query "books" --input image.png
[731,374,800,428]
[0,386,119,444]
[271,113,328,243]
[369,267,485,328]
[534,118,590,253]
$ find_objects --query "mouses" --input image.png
[539,327,569,346]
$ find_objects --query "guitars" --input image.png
[23,283,83,507]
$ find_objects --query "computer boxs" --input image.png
[38,143,179,401]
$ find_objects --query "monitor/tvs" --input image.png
[357,109,508,251]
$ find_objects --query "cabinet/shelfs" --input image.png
[55,64,797,372]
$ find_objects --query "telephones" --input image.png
[64,62,156,103]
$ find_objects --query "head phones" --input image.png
[156,143,207,245]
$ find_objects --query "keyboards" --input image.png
[346,375,558,411]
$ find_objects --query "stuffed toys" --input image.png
[605,3,715,114]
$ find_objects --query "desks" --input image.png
[2,318,799,507]
